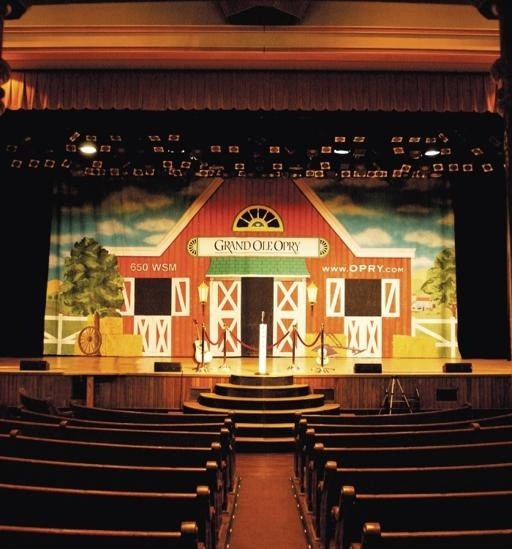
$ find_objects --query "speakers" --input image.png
[442,362,473,373]
[354,364,382,374]
[154,362,181,372]
[20,360,50,370]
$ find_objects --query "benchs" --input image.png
[288,400,512,549]
[0,384,241,549]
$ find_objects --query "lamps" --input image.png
[332,133,351,155]
[76,131,98,157]
[421,142,441,157]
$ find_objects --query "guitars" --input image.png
[316,328,331,366]
[191,319,213,364]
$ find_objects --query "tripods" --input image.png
[378,376,412,414]
